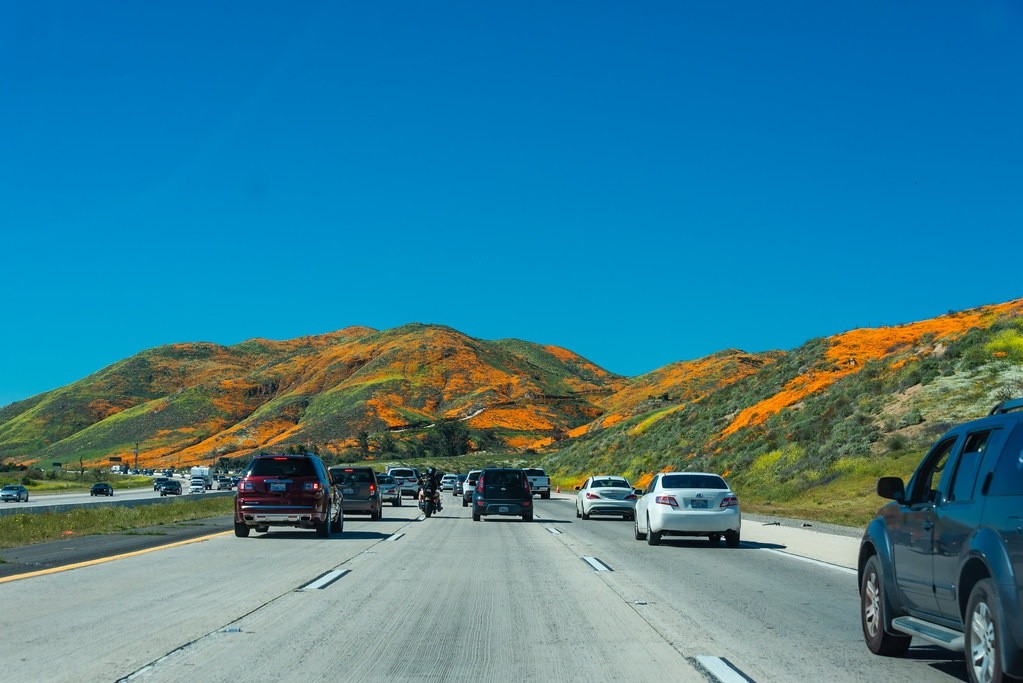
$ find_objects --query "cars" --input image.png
[0,485,29,503]
[574,475,637,521]
[376,472,405,506]
[414,471,470,497]
[112,464,244,496]
[90,483,113,497]
[634,472,742,550]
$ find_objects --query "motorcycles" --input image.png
[417,481,444,518]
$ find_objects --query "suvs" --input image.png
[518,468,551,500]
[328,465,382,519]
[461,469,486,506]
[852,395,1022,683]
[233,450,346,538]
[388,467,420,499]
[471,468,533,522]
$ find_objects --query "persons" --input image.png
[418,466,442,512]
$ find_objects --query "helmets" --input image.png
[426,466,436,476]
